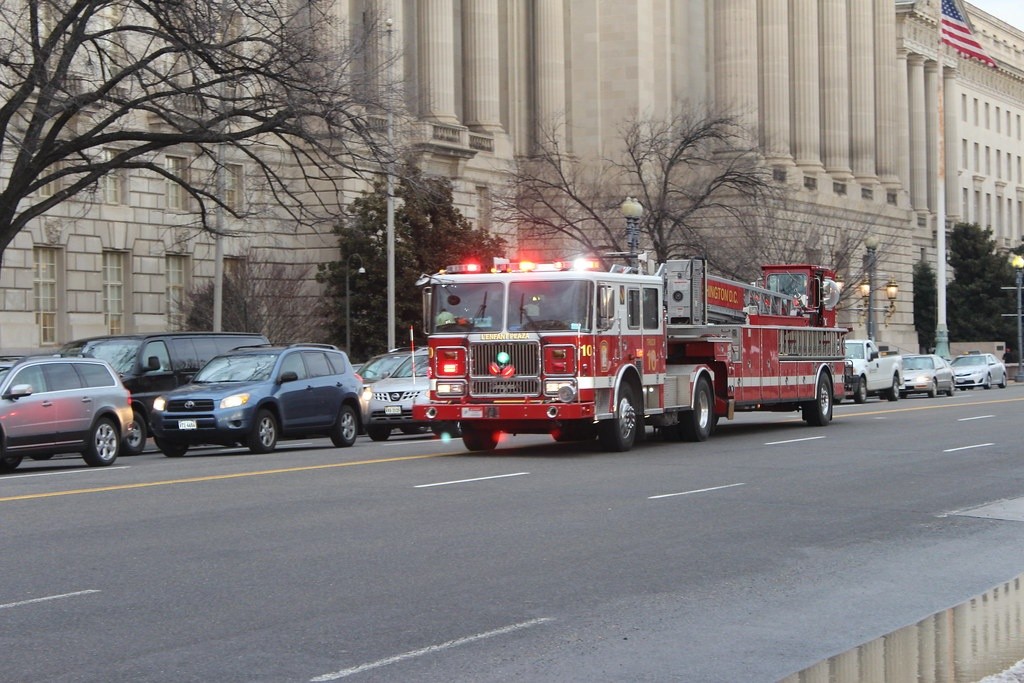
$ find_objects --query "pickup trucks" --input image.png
[833,340,903,406]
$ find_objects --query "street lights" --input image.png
[1012,256,1023,382]
[865,237,898,341]
[834,273,870,328]
[346,253,366,360]
[622,197,643,275]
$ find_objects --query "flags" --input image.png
[941,0,998,68]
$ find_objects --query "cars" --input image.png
[949,353,1007,389]
[897,354,955,398]
[356,351,413,386]
[360,348,463,441]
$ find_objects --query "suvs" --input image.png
[0,353,134,475]
[152,343,365,453]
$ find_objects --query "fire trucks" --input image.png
[409,251,844,452]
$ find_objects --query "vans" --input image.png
[54,332,273,455]
